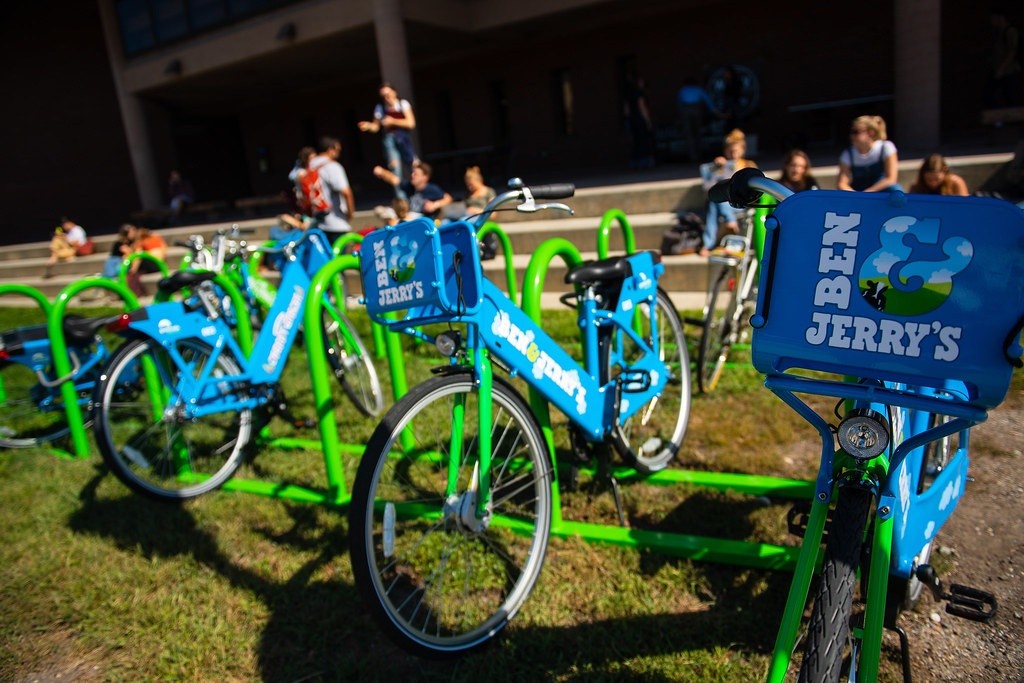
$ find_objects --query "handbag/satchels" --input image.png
[660,213,702,253]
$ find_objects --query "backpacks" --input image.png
[295,167,332,214]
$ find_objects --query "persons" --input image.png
[285,84,498,261]
[696,115,969,257]
[42,219,168,305]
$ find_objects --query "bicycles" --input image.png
[702,158,1022,683]
[0,209,381,504]
[683,173,793,394]
[333,176,693,658]
